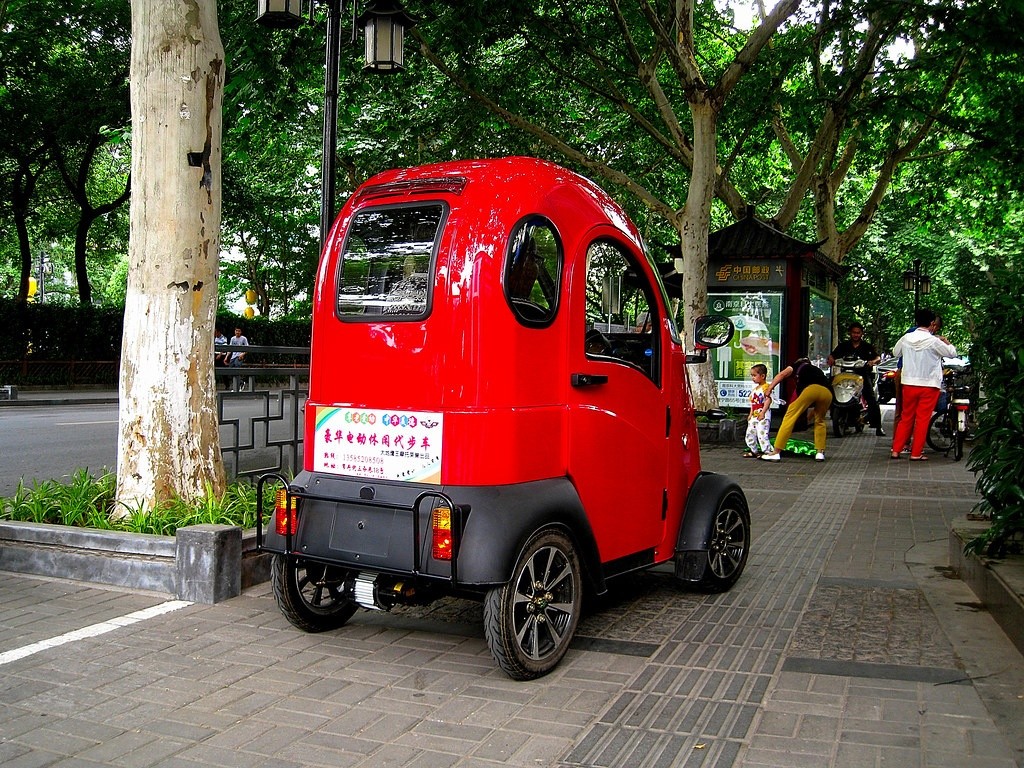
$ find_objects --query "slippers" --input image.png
[891,452,901,459]
[909,455,930,461]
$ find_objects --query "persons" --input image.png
[214,327,250,392]
[827,323,886,437]
[739,337,779,356]
[743,357,834,463]
[893,308,957,461]
[635,312,652,332]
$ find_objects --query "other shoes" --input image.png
[815,452,825,461]
[225,387,229,390]
[240,382,246,390]
[761,453,780,462]
[231,389,233,393]
[902,448,911,453]
[875,428,887,436]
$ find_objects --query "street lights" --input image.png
[253,0,414,265]
[903,258,932,327]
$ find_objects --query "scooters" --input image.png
[828,358,880,438]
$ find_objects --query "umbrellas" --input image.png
[728,313,768,348]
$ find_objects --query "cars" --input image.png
[254,158,751,682]
[341,285,387,301]
[877,358,900,404]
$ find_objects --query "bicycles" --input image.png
[926,371,972,460]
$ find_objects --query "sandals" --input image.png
[756,454,761,459]
[743,453,760,458]
